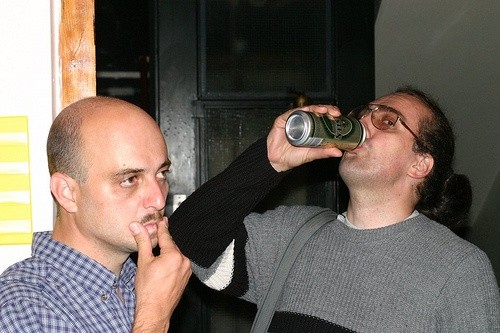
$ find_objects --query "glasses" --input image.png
[348,103,418,140]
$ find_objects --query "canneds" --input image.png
[284,109,367,151]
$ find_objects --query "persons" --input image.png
[168,88,500,333]
[0,96,193,333]
